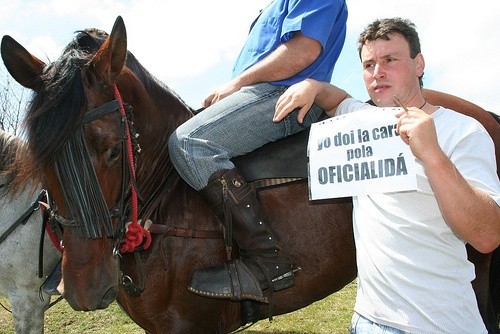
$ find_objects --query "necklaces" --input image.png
[418,101,428,110]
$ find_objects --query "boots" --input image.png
[189,166,296,297]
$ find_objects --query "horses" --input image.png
[0,15,500,334]
[0,130,62,334]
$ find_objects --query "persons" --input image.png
[168,0,350,292]
[273,18,500,334]
